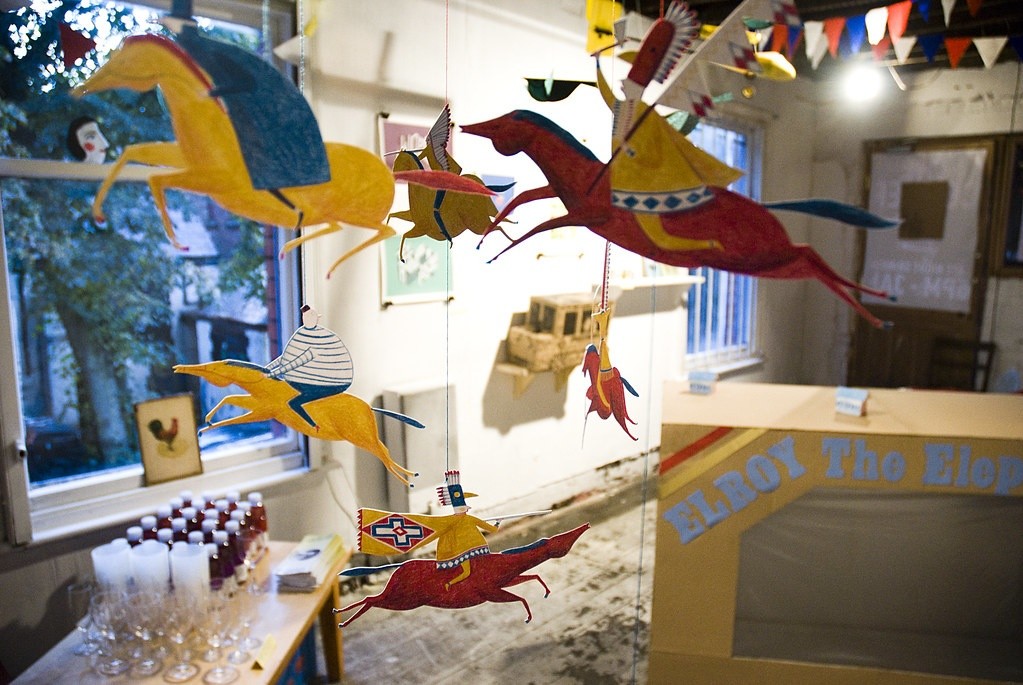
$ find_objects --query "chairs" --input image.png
[926,336,996,394]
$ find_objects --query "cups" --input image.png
[91,543,210,595]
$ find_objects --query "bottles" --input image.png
[112,490,269,598]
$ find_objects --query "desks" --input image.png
[4,538,353,685]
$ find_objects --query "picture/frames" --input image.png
[134,392,203,487]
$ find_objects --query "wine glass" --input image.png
[69,571,262,685]
[235,529,269,595]
